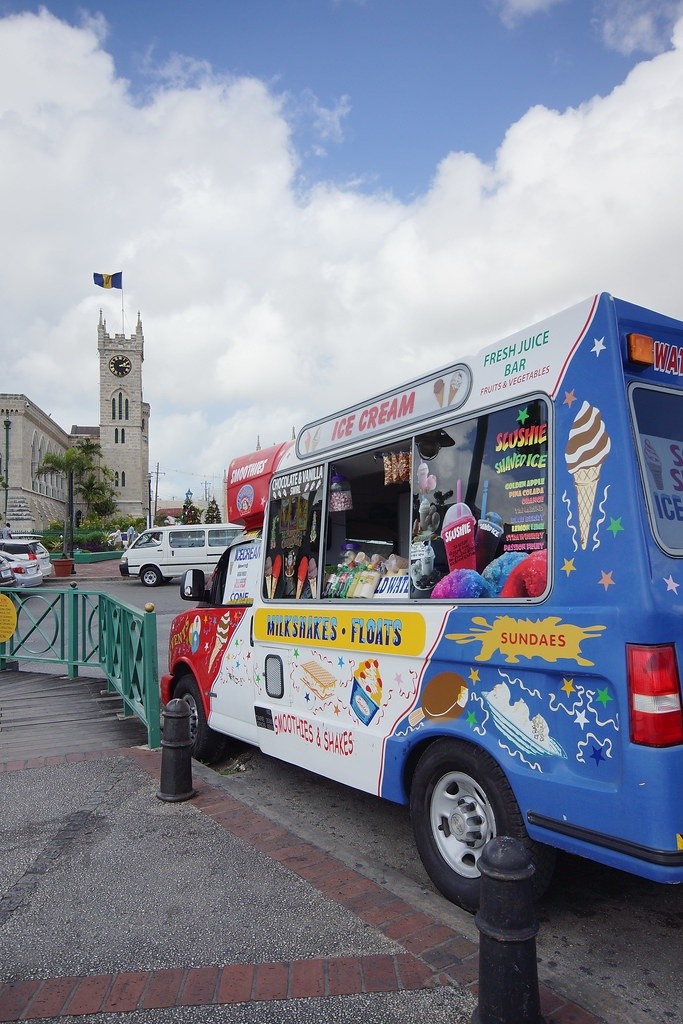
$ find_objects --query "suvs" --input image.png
[0,539,53,579]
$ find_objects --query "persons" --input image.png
[59,532,64,542]
[2,523,12,539]
[127,523,134,548]
[111,525,122,550]
[152,532,159,541]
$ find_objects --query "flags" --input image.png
[94,272,122,289]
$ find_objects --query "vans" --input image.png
[119,522,252,587]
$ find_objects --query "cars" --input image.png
[0,550,43,587]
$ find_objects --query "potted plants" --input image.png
[51,553,75,577]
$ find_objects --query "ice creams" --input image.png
[433,372,463,408]
[263,554,282,599]
[295,557,318,599]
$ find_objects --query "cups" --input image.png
[441,517,476,571]
[473,519,504,575]
[419,510,430,530]
[422,557,434,575]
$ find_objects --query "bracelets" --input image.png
[112,538,115,540]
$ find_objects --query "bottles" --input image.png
[322,543,381,599]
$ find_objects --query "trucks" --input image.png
[153,289,683,916]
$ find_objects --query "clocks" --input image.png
[108,355,132,378]
